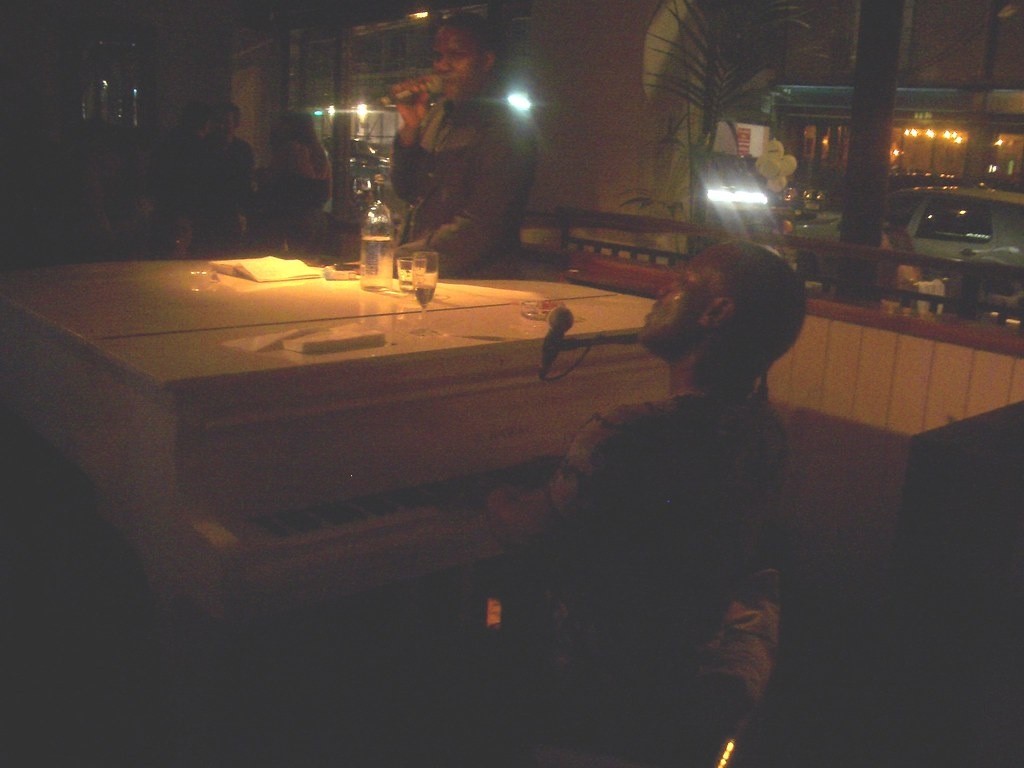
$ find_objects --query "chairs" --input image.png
[532,570,783,768]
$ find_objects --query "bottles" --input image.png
[361,172,392,292]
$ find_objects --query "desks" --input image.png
[1,257,686,601]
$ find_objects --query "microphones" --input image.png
[536,307,574,379]
[367,75,444,110]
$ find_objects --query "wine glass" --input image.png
[409,252,441,337]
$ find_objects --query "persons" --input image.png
[180,105,380,257]
[391,11,531,280]
[484,240,808,763]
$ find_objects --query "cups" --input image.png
[397,255,427,293]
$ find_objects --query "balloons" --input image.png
[754,141,798,192]
